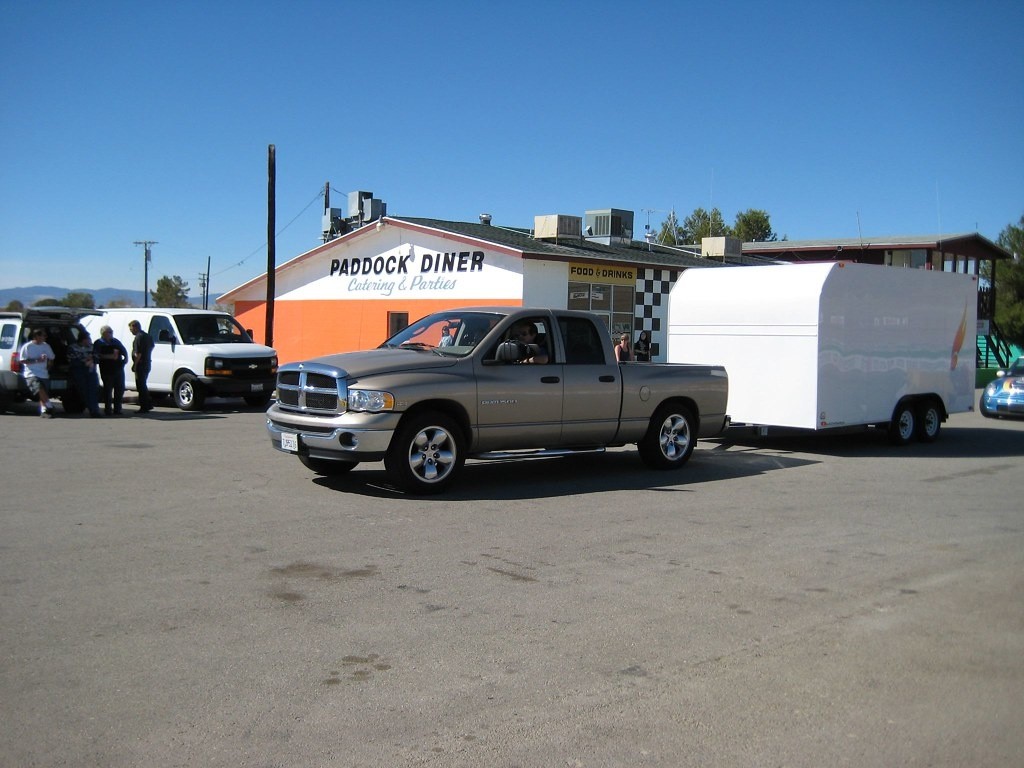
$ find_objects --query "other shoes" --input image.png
[104,409,122,415]
[90,411,103,417]
[135,406,153,414]
[40,408,55,418]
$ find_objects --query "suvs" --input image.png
[0,307,108,414]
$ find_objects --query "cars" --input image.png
[979,357,1024,420]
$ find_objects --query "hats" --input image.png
[620,333,629,340]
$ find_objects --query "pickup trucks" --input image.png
[267,304,731,496]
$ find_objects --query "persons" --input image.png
[222,319,231,334]
[517,321,549,363]
[20,329,57,419]
[66,331,105,419]
[92,326,128,416]
[129,320,155,413]
[438,325,453,347]
[634,330,652,361]
[614,333,634,361]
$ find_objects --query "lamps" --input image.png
[376,214,385,231]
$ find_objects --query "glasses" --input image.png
[518,330,530,336]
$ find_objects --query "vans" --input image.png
[77,307,279,410]
